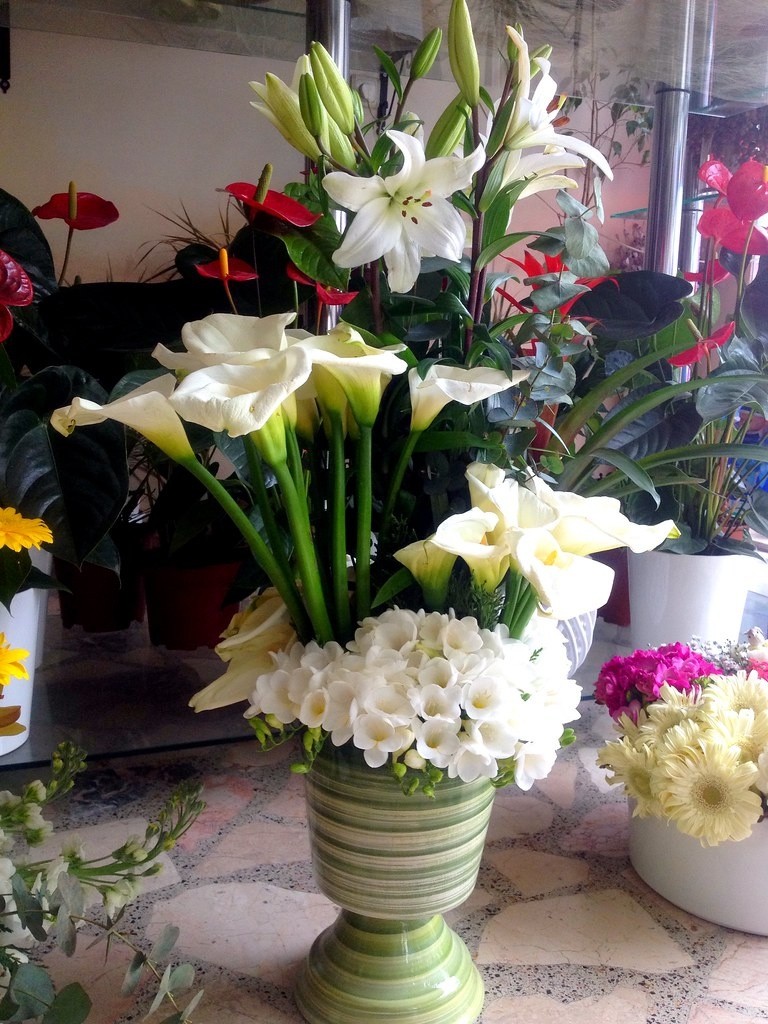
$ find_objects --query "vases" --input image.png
[628,783,768,938]
[293,730,497,1024]
[0,546,54,759]
[145,560,240,652]
[54,530,161,633]
[627,545,756,655]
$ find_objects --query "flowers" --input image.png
[0,0,768,1024]
